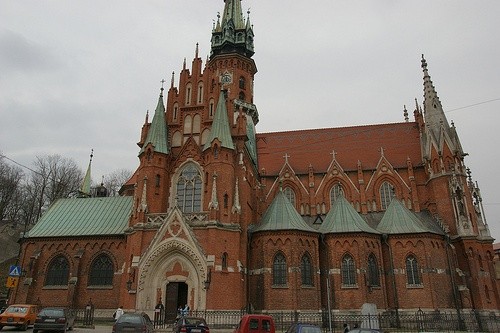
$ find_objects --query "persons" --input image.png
[153,300,164,325]
[176,304,190,326]
[112,305,124,323]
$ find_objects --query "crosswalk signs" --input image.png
[8,266,21,277]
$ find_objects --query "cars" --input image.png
[111,312,154,333]
[286,323,321,333]
[173,317,210,333]
[32,307,76,333]
[345,327,384,333]
[0,304,40,331]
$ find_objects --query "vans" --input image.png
[234,315,275,333]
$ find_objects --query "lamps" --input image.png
[30,255,39,259]
[73,254,81,258]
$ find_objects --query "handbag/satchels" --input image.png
[177,310,180,314]
[113,313,116,318]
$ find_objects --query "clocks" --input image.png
[222,75,231,83]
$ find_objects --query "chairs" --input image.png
[196,321,201,324]
[185,321,191,324]
